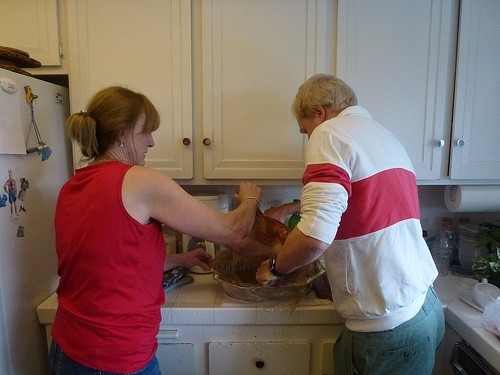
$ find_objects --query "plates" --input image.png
[457,290,484,313]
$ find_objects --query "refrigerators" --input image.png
[0,69,73,375]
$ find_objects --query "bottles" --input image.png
[431,217,471,277]
[288,199,301,228]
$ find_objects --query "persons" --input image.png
[51,86,263,375]
[256,73,445,375]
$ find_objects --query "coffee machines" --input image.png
[177,190,229,273]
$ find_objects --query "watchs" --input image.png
[269,255,283,277]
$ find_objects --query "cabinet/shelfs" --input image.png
[65,0,326,185]
[336,0,500,186]
[45,323,340,375]
[0,0,63,75]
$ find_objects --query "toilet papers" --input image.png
[443,185,500,213]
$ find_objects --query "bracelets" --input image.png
[246,197,259,205]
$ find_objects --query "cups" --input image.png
[472,277,500,307]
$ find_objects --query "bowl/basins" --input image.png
[212,264,325,301]
